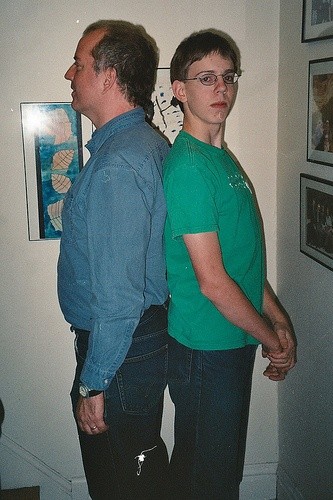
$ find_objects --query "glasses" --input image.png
[183,71,240,87]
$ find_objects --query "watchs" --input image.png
[77,381,105,399]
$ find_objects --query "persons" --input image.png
[161,31,297,500]
[56,19,172,500]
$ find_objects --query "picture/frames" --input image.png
[307,56,333,167]
[301,0,333,43]
[299,172,333,273]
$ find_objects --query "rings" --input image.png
[91,425,97,430]
